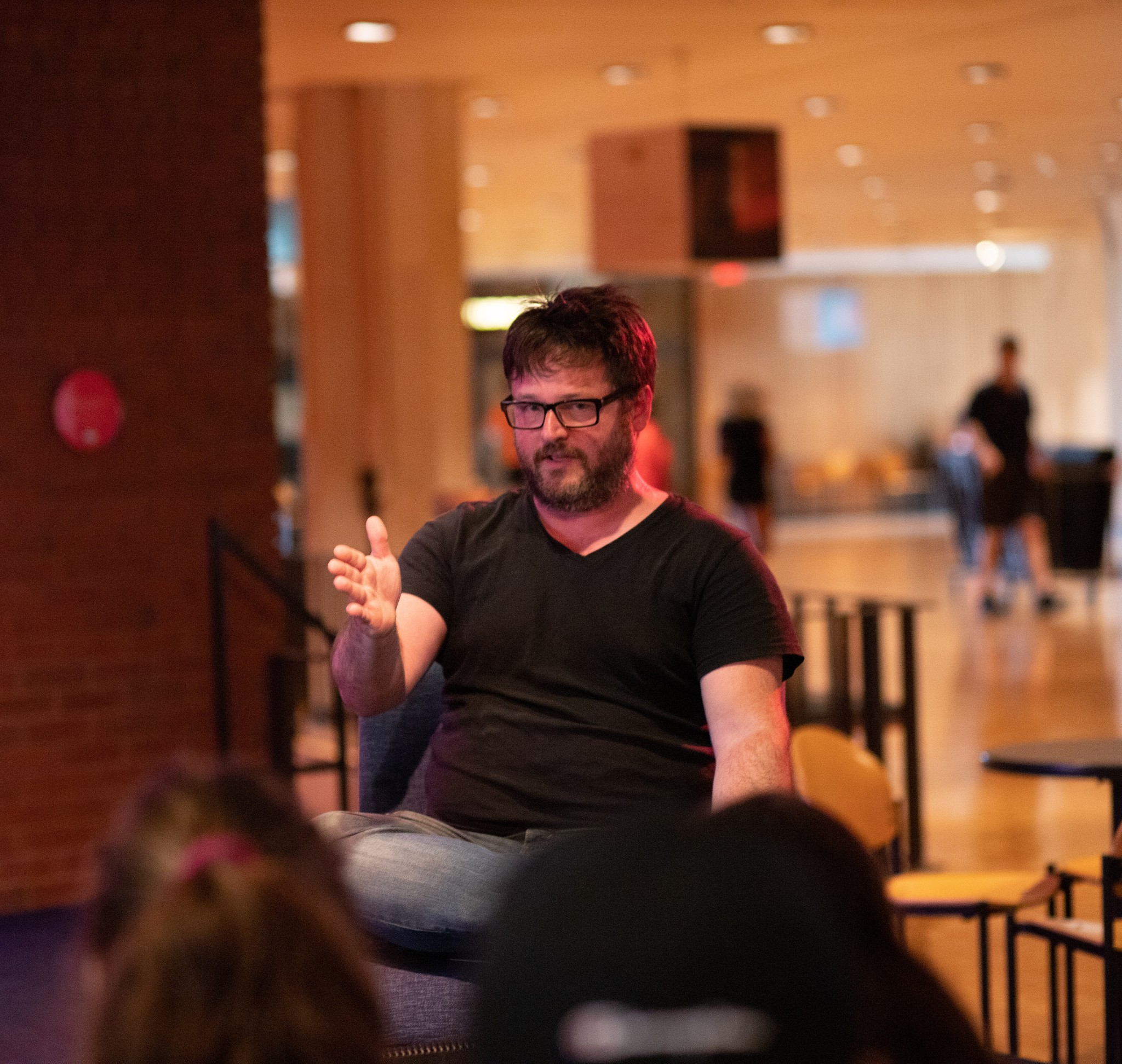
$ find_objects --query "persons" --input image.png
[715,384,773,557]
[955,336,1057,617]
[77,748,991,1064]
[458,361,685,501]
[327,280,804,936]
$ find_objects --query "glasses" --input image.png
[500,383,640,430]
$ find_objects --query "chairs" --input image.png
[1006,818,1122,1064]
[787,721,1064,1064]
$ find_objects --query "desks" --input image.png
[975,734,1122,1064]
